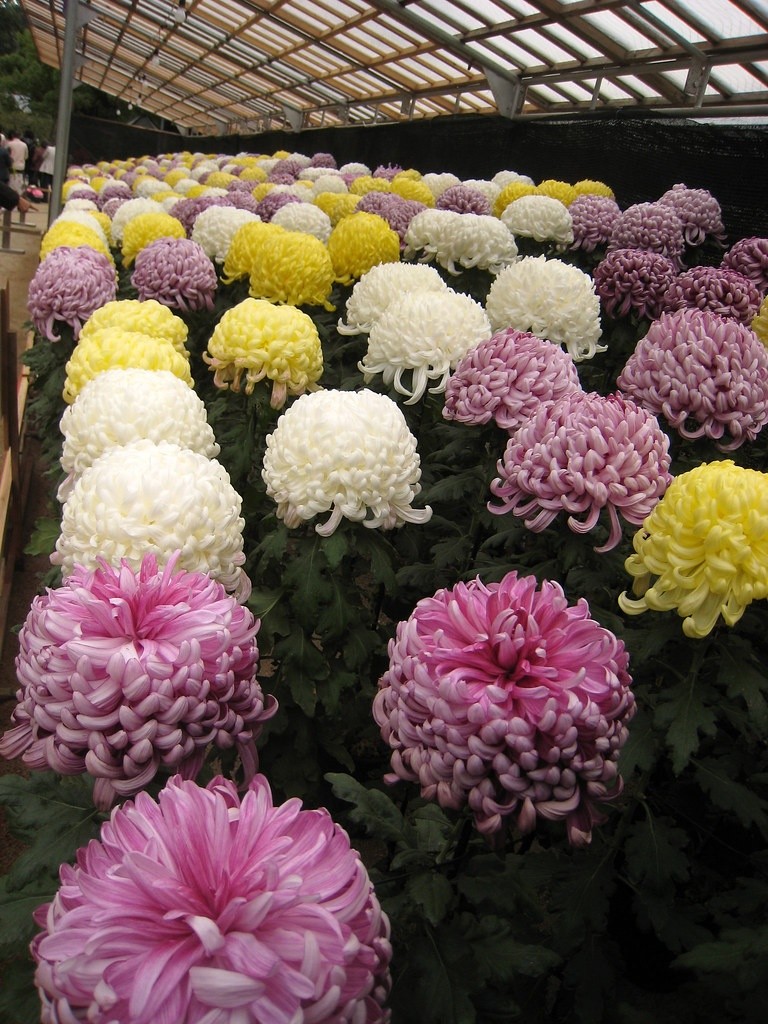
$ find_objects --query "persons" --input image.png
[0,127,56,213]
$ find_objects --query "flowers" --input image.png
[0,149,768,1024]
